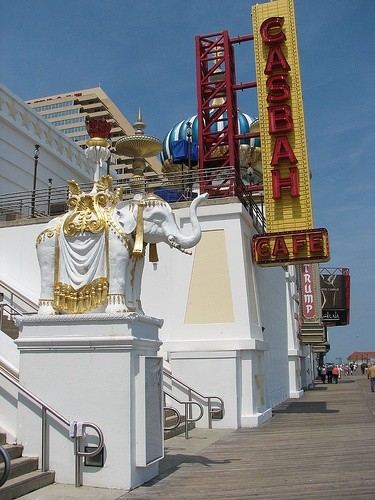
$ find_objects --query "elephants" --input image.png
[36,192,210,316]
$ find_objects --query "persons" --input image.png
[367,361,375,392]
[317,361,375,384]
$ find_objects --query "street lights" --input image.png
[247,162,253,218]
[185,121,193,201]
[107,137,112,175]
[30,144,40,218]
[47,177,52,214]
[259,190,264,235]
